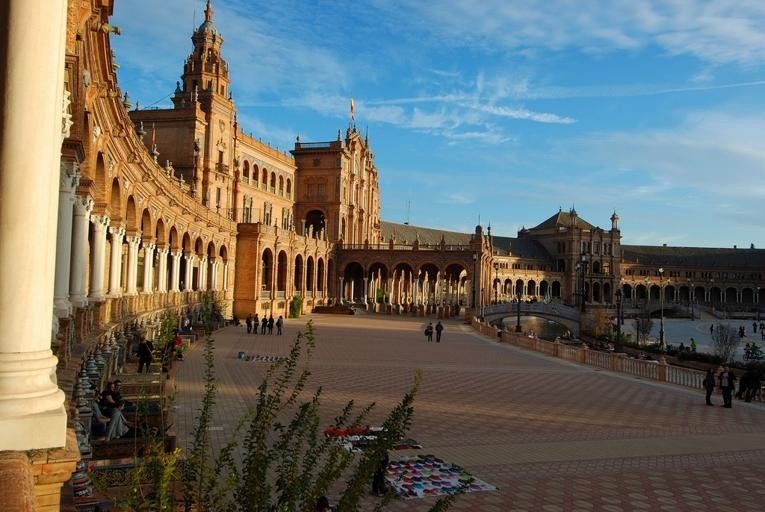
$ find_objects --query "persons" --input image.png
[737,319,764,360]
[426,323,433,342]
[135,336,151,373]
[434,321,443,342]
[689,337,696,351]
[172,332,182,345]
[316,496,331,512]
[701,362,763,409]
[246,313,285,336]
[710,324,713,334]
[678,342,684,352]
[87,376,159,443]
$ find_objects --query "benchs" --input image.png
[67,309,232,512]
